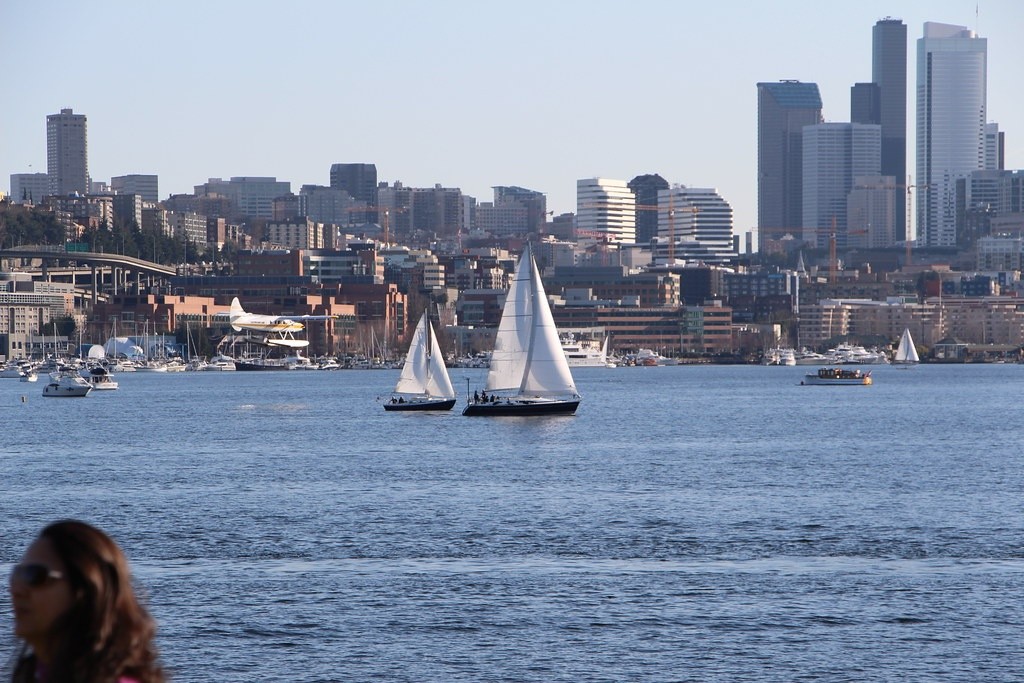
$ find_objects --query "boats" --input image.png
[801,367,872,385]
[42,371,93,396]
[761,341,895,366]
[79,362,118,390]
[558,330,680,368]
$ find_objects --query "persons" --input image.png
[8,520,167,683]
[474,389,494,404]
[391,396,404,404]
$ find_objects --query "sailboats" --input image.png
[0,318,492,382]
[382,308,458,410]
[461,240,581,415]
[894,328,919,365]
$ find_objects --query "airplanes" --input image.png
[230,296,310,347]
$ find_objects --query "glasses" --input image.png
[10,564,71,590]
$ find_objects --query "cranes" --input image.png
[542,211,554,237]
[347,206,406,246]
[574,229,615,265]
[856,174,928,266]
[752,217,868,282]
[583,194,702,264]
